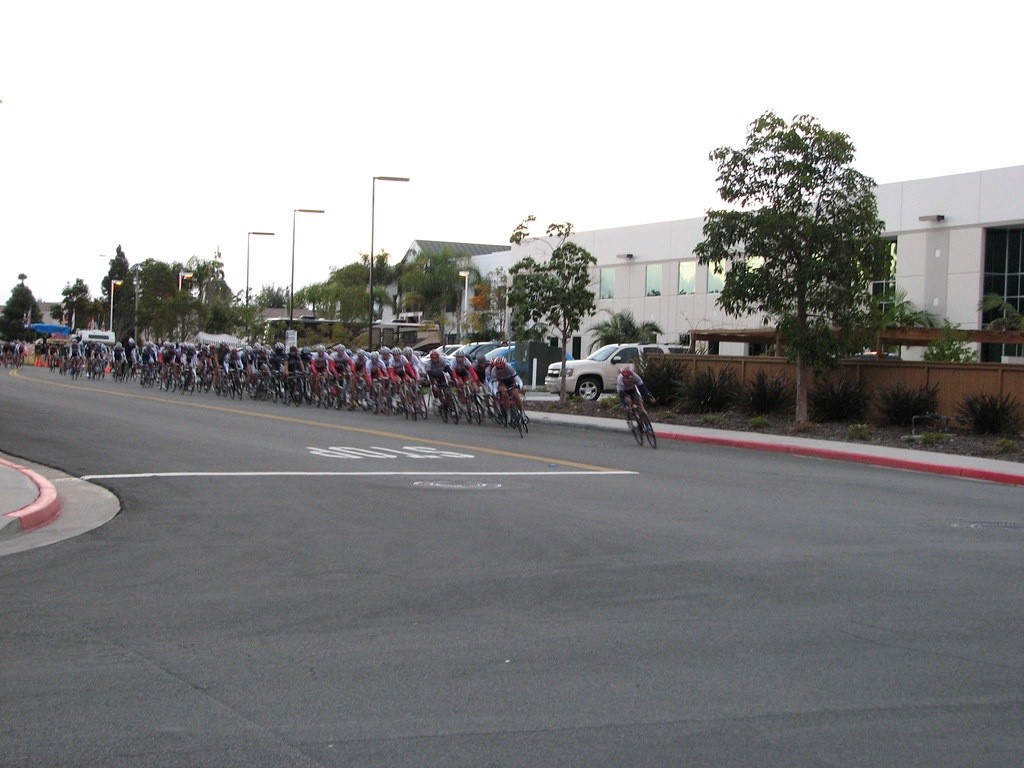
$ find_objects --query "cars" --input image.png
[420,343,463,369]
[483,343,574,386]
[446,341,518,378]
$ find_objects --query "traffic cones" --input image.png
[106,361,110,372]
[38,357,43,367]
[45,356,50,368]
[34,356,38,367]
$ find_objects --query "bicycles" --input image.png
[0,350,27,370]
[619,391,659,450]
[45,349,531,440]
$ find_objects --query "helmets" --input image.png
[620,365,634,375]
[0,338,509,370]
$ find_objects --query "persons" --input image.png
[616,366,655,419]
[0,338,525,428]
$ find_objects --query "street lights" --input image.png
[290,209,325,332]
[459,270,470,339]
[178,272,194,343]
[368,176,410,354]
[109,280,124,333]
[246,232,275,340]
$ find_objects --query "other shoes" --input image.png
[646,423,651,432]
[627,417,634,430]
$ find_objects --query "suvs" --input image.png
[544,343,690,403]
